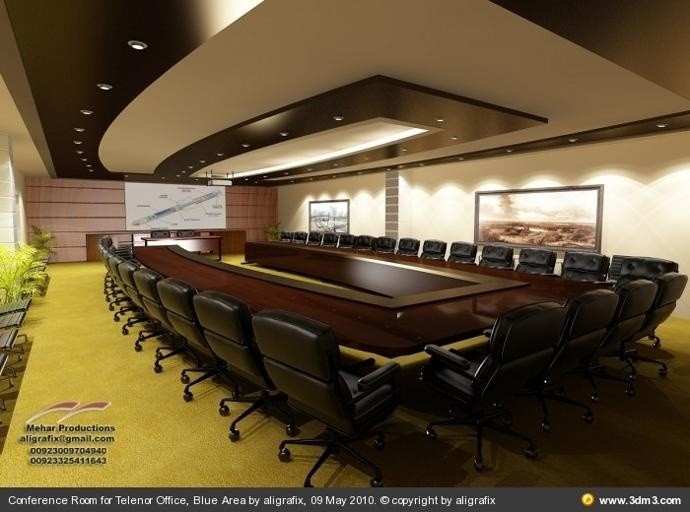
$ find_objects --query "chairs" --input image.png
[0,257,49,412]
[97,231,688,488]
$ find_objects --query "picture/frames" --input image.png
[474,184,604,255]
[308,199,349,233]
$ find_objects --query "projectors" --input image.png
[207,179,233,186]
